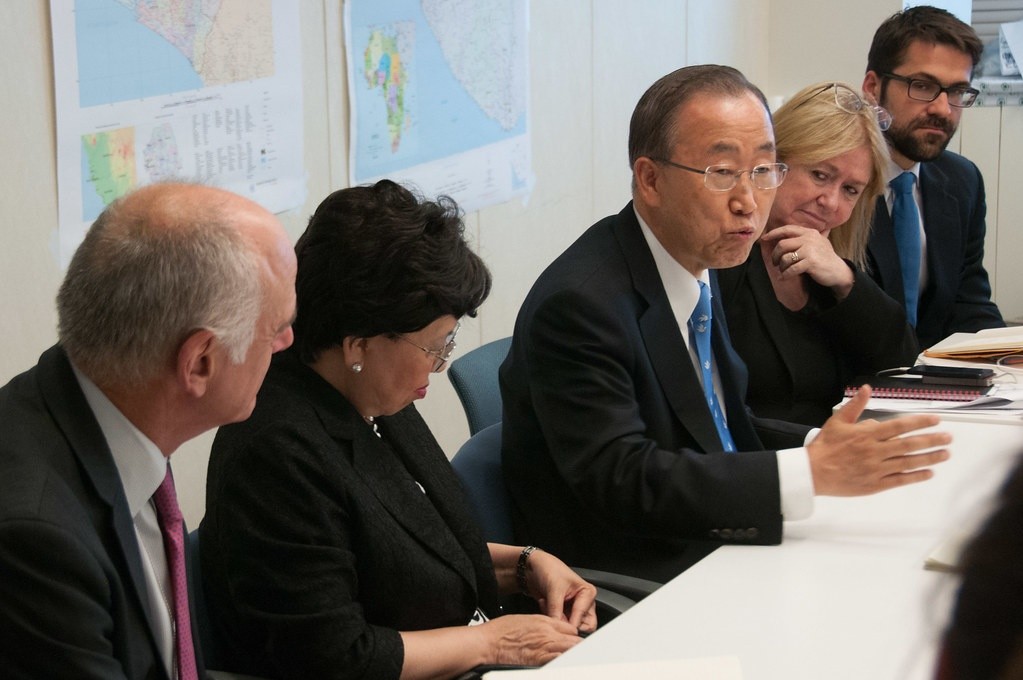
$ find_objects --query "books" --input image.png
[843,325,1023,401]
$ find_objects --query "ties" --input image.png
[152,462,198,680]
[689,279,737,453]
[888,170,922,328]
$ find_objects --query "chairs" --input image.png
[447,336,513,438]
[451,421,664,600]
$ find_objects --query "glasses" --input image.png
[654,158,790,193]
[791,81,893,131]
[875,71,980,109]
[382,322,460,373]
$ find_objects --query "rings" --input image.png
[791,251,799,262]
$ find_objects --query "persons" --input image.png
[498,64,953,600]
[197,179,597,680]
[0,181,298,680]
[861,5,1005,350]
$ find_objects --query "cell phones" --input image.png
[906,364,994,379]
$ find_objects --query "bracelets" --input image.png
[516,545,543,594]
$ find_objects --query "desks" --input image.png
[528,362,1023,680]
